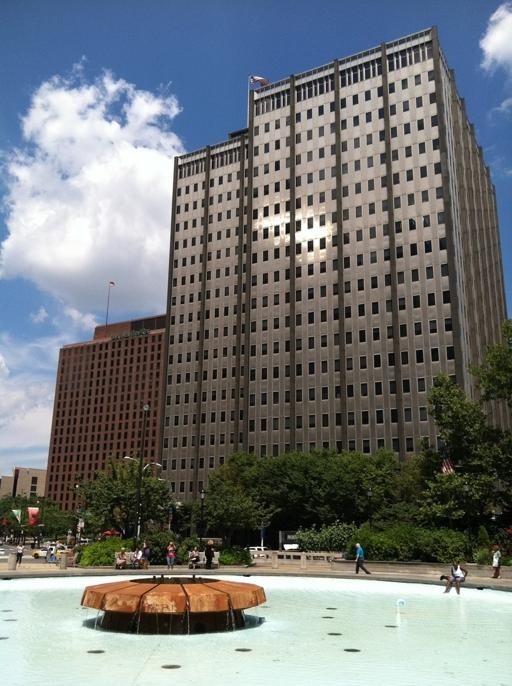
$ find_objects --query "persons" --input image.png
[190,546,200,570]
[491,545,502,579]
[444,561,468,594]
[167,542,176,569]
[355,543,372,574]
[46,548,56,563]
[132,547,142,569]
[141,542,151,570]
[16,542,24,565]
[205,540,214,569]
[116,548,127,569]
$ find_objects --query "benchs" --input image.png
[188,551,219,569]
[115,552,145,569]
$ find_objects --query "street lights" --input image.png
[124,405,162,542]
[199,489,207,543]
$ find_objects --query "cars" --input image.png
[244,547,269,551]
[32,543,66,559]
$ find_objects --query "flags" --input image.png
[28,507,39,526]
[251,76,268,86]
